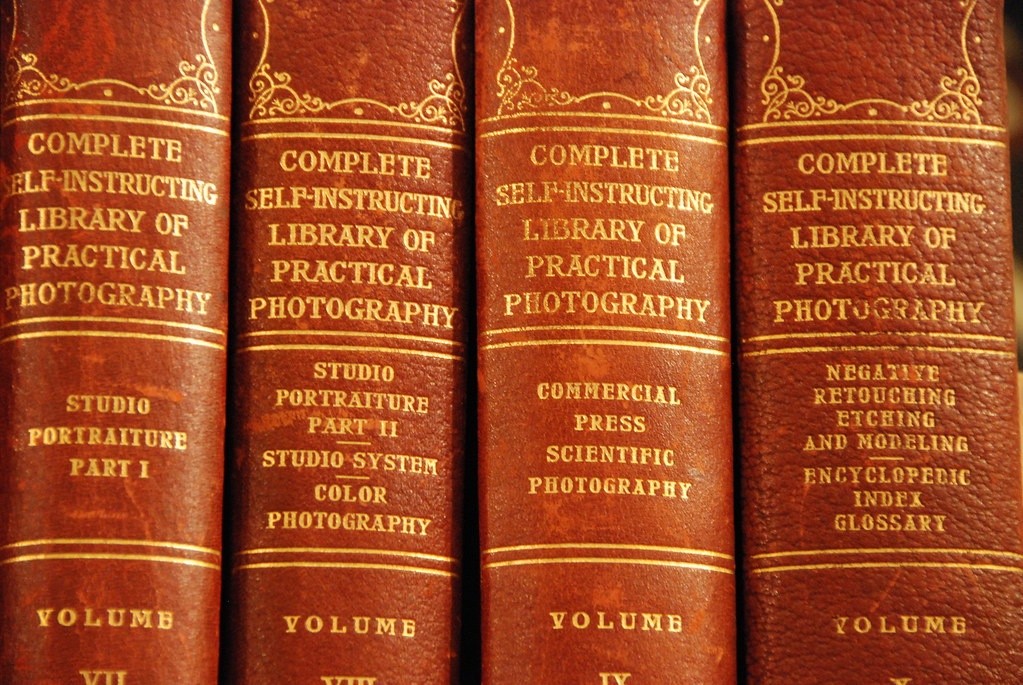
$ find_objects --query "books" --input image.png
[0,0,1023,684]
